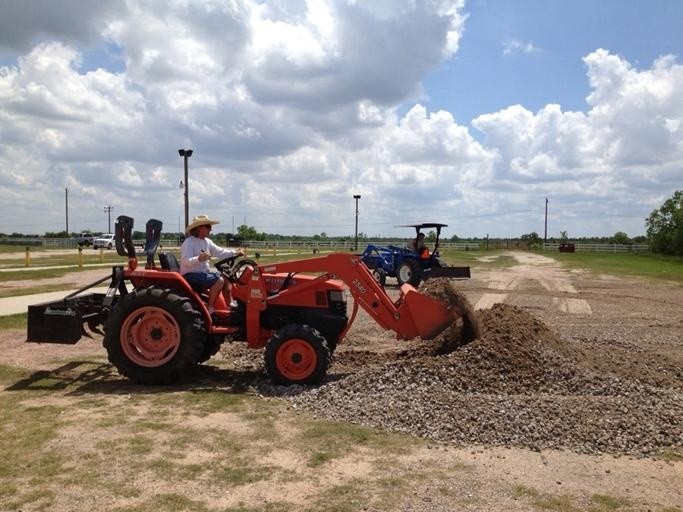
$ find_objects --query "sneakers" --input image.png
[210,314,222,322]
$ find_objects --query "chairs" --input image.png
[158,252,203,294]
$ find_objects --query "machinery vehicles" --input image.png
[25,213,465,389]
[354,224,475,290]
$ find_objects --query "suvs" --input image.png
[94,234,116,250]
[77,233,98,246]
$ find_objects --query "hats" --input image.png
[185,215,219,233]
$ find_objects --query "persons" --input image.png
[409,233,426,253]
[179,214,245,325]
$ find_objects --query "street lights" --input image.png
[103,205,115,233]
[65,185,70,236]
[176,148,192,237]
[352,192,362,251]
[543,196,549,242]
